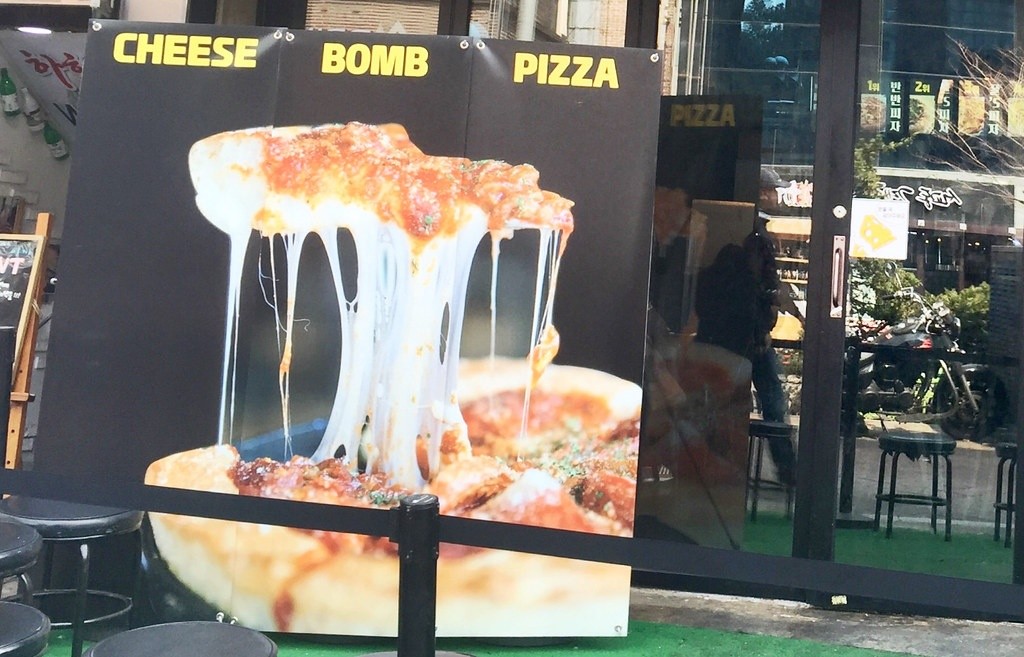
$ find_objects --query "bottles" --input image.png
[44,120,69,160]
[0,68,21,116]
[21,87,45,132]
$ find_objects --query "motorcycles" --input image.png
[834,262,1008,442]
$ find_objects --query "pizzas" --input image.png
[144,119,643,636]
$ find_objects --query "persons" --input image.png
[693,170,798,485]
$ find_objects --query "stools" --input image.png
[744,420,798,521]
[0,495,280,657]
[993,442,1020,546]
[873,430,957,541]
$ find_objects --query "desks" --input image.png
[753,339,1020,532]
